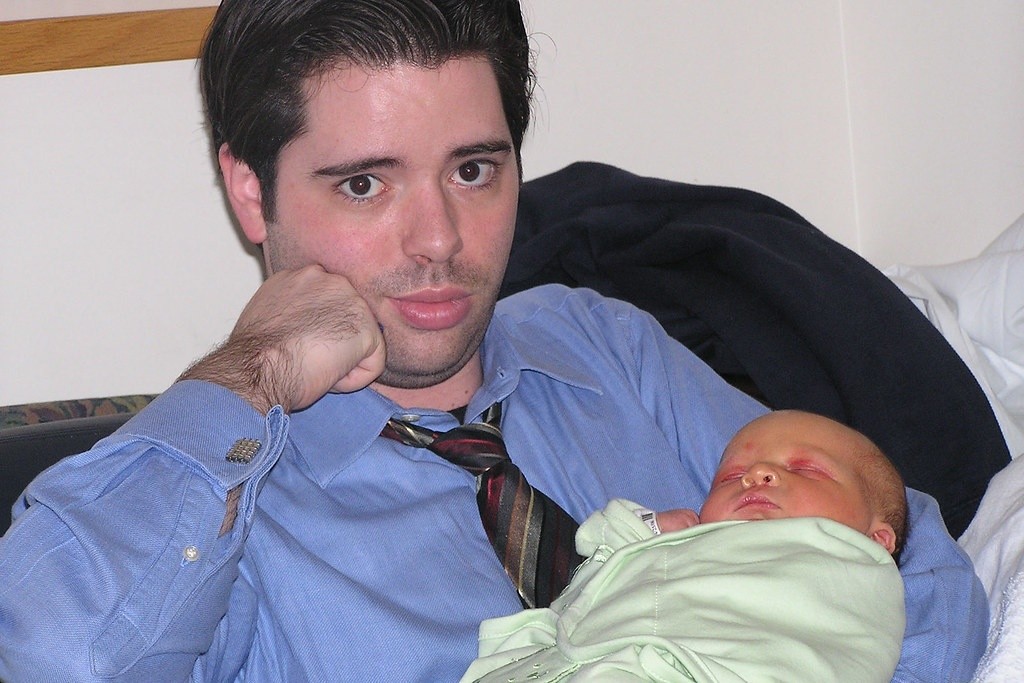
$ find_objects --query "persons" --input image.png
[460,411,909,681]
[0,0,989,683]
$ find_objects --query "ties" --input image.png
[380,394,588,610]
